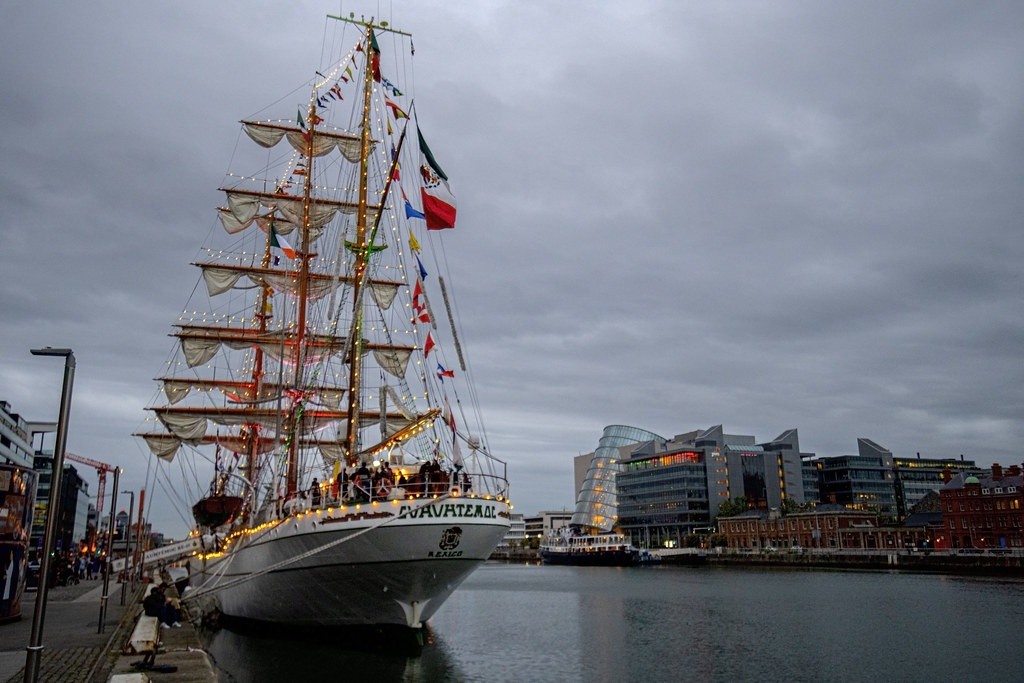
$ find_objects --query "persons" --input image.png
[307,461,470,506]
[0,550,14,618]
[54,550,114,582]
[143,581,183,628]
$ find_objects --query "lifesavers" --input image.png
[376,478,392,496]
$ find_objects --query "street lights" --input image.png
[95,465,120,634]
[120,491,135,606]
[24,346,76,683]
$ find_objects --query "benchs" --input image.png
[125,583,157,670]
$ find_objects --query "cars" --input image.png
[27,561,81,587]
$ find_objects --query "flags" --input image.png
[227,28,462,470]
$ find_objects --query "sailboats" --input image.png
[130,7,512,628]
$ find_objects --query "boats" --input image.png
[538,525,641,565]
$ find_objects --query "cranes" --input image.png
[64,451,120,511]
